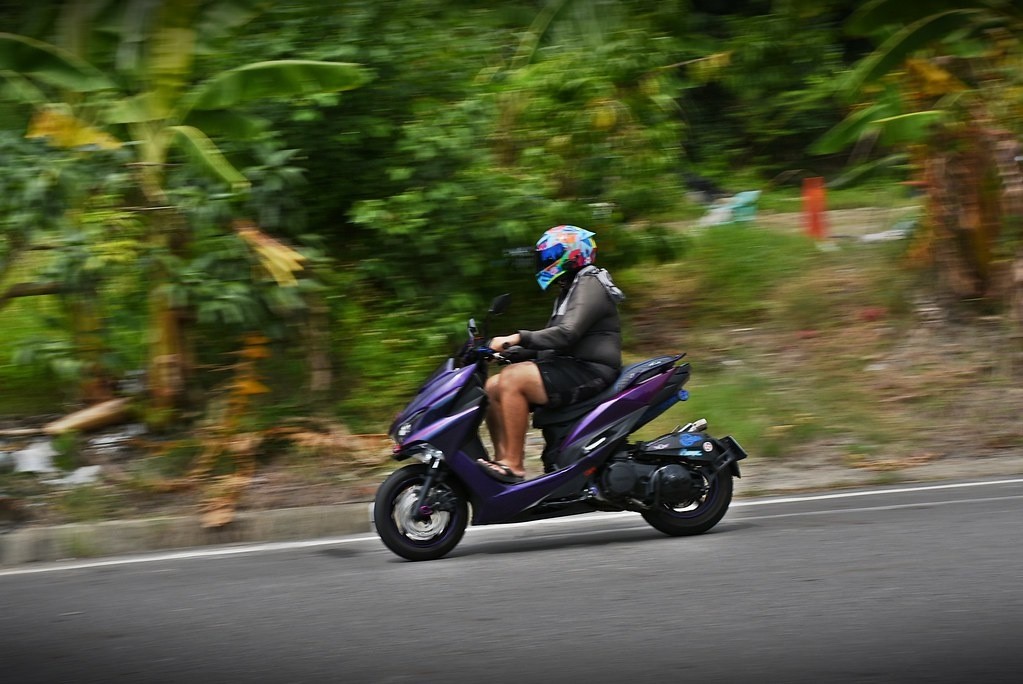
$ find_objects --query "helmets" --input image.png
[533,225,598,290]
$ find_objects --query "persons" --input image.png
[475,225,622,484]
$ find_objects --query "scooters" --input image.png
[374,292,748,560]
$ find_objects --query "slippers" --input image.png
[477,462,524,482]
